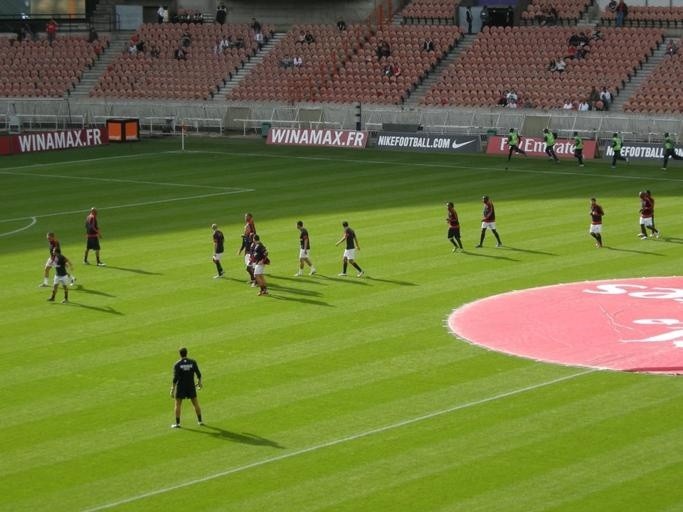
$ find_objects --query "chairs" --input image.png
[1,37,112,97]
[602,4,683,29]
[624,34,683,114]
[418,26,666,111]
[400,0,463,24]
[518,0,593,25]
[89,22,277,101]
[226,23,465,106]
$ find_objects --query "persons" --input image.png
[336,222,364,278]
[169,348,204,428]
[638,190,661,244]
[36,206,106,304]
[505,128,528,163]
[211,224,225,280]
[542,128,560,164]
[277,15,436,79]
[295,221,316,277]
[120,3,263,62]
[573,131,585,167]
[237,213,270,297]
[445,202,464,253]
[589,198,604,249]
[609,133,629,169]
[661,133,683,171]
[475,195,502,248]
[466,0,629,112]
[8,17,100,53]
[665,39,677,56]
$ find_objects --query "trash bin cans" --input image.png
[261,122,271,136]
